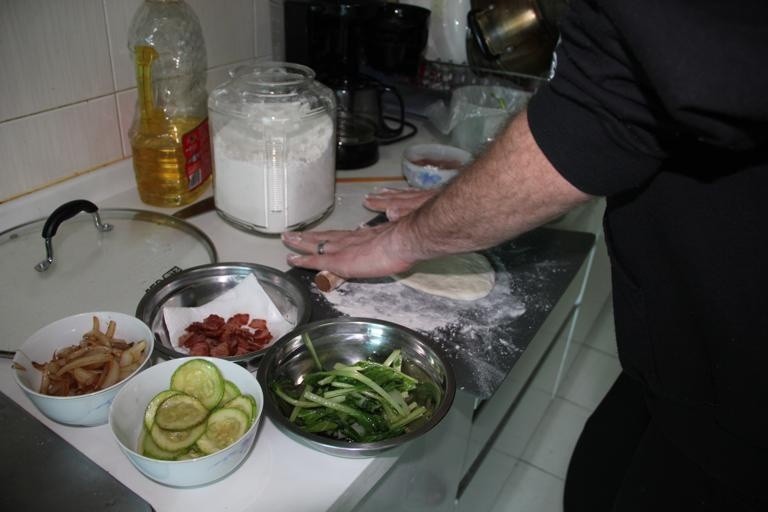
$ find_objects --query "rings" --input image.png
[318,241,327,255]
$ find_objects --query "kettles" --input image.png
[329,73,404,148]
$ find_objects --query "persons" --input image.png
[280,0,768,512]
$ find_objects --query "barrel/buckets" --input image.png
[128,0,213,210]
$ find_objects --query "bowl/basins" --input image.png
[401,142,473,191]
[108,356,265,488]
[12,311,155,427]
[256,316,456,460]
[135,262,309,374]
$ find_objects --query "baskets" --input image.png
[418,54,559,104]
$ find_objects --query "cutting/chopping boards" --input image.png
[283,212,596,402]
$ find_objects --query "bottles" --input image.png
[208,62,334,240]
